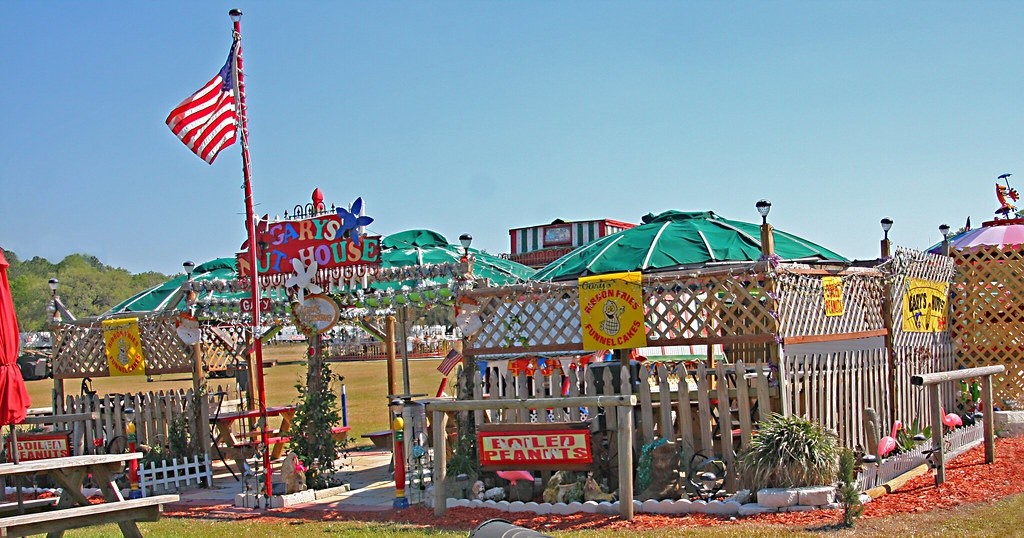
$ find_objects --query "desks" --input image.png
[208,406,308,474]
[0,452,148,538]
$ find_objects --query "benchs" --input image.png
[0,498,57,511]
[0,494,180,532]
[231,430,279,437]
[255,427,351,449]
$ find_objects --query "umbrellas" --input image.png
[107,257,285,315]
[925,225,1024,254]
[0,250,31,515]
[332,231,536,285]
[527,210,851,389]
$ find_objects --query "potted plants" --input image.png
[743,414,832,486]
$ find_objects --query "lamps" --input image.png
[938,224,949,238]
[182,260,195,279]
[881,217,893,238]
[48,278,58,294]
[458,232,472,255]
[755,199,772,224]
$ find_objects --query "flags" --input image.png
[437,349,463,376]
[165,39,240,165]
